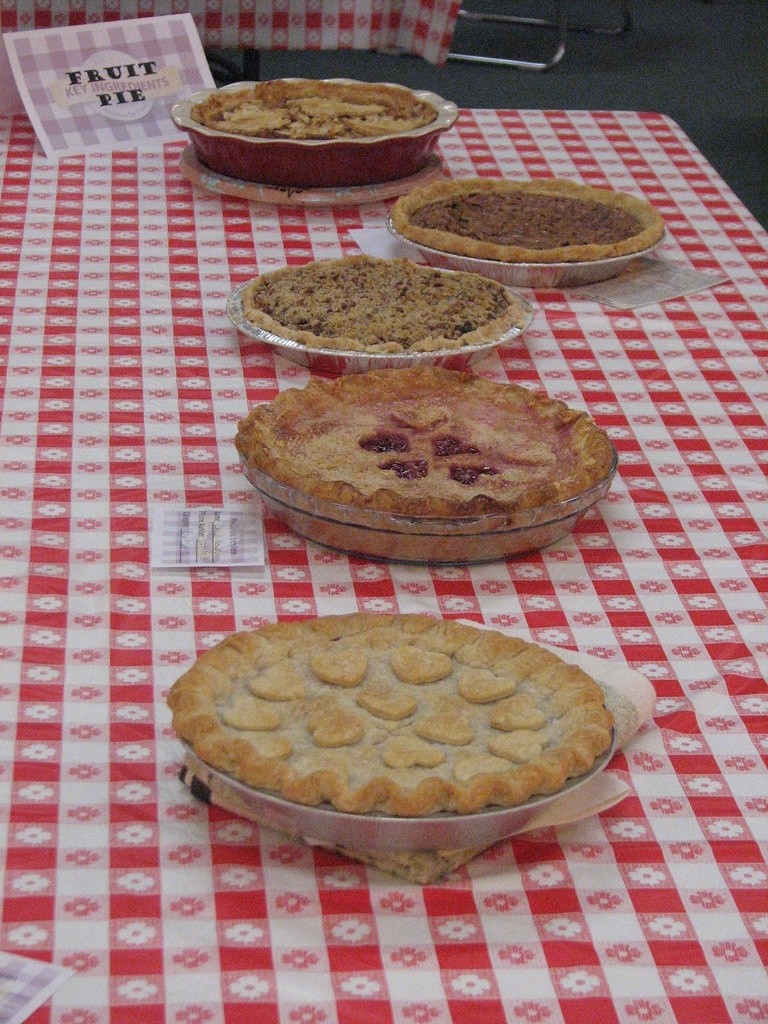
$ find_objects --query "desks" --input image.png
[1,106,768,1024]
[0,0,639,73]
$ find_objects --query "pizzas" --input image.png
[390,178,666,263]
[164,613,614,815]
[241,255,530,354]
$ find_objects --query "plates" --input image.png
[384,211,665,287]
[236,431,619,566]
[227,266,533,374]
[171,77,459,189]
[174,620,657,853]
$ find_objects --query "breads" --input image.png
[191,80,440,139]
[234,365,616,564]
